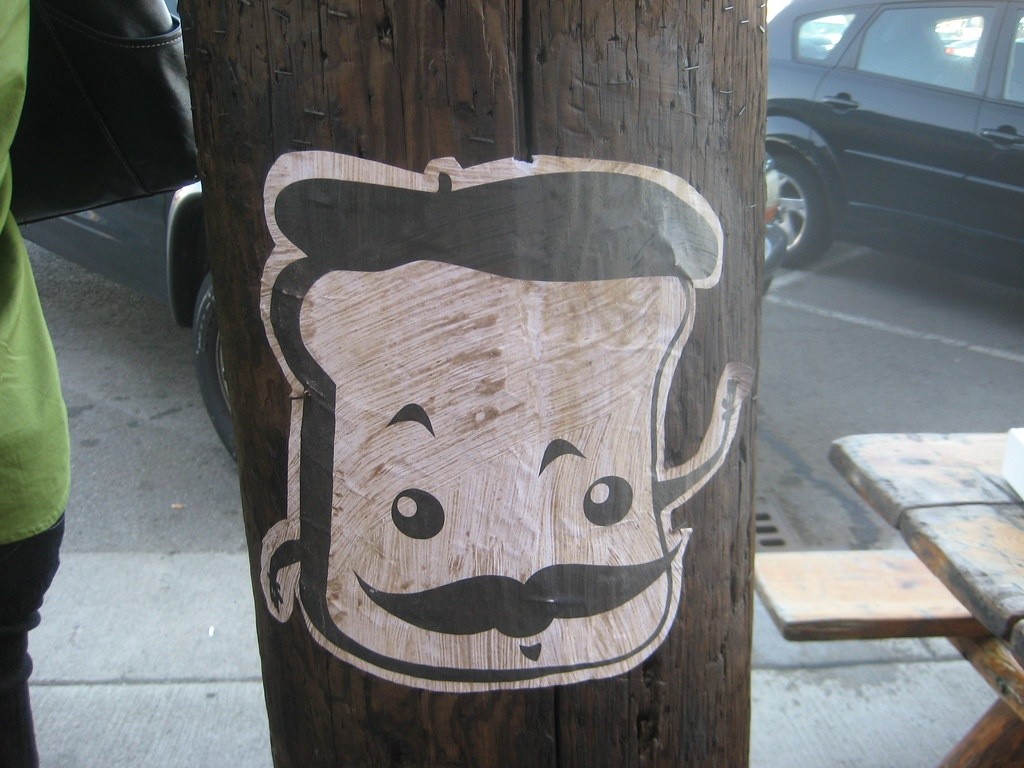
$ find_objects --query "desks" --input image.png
[826,429,1024,768]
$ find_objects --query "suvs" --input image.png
[766,0,1024,285]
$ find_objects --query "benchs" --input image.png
[755,551,1024,643]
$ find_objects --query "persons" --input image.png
[0,0,74,768]
